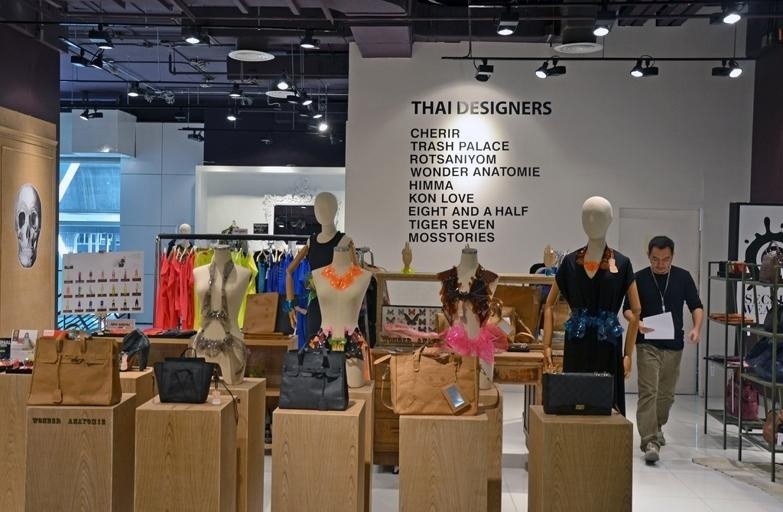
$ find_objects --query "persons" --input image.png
[285,192,358,351]
[437,248,500,390]
[311,247,372,388]
[176,223,195,253]
[626,236,704,466]
[192,245,253,385]
[542,197,641,416]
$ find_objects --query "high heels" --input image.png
[119,329,148,371]
[137,328,150,368]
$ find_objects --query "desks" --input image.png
[369,347,544,474]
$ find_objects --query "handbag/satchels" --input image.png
[759,241,783,284]
[764,296,783,333]
[746,336,783,383]
[374,337,500,415]
[544,372,614,416]
[727,378,760,421]
[279,334,349,410]
[153,348,214,404]
[26,324,122,405]
[763,409,783,444]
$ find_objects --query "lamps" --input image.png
[475,74,490,82]
[128,82,140,97]
[721,0,742,24]
[185,32,200,44]
[630,61,644,77]
[535,62,549,79]
[188,134,204,142]
[727,60,742,79]
[80,109,89,121]
[91,54,103,69]
[299,31,315,49]
[496,26,516,36]
[592,25,609,37]
[96,44,113,50]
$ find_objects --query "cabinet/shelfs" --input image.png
[737,262,783,482]
[375,272,556,346]
[704,259,743,449]
[84,337,293,450]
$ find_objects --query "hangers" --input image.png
[158,240,300,262]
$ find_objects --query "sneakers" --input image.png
[645,443,659,461]
[658,431,665,445]
